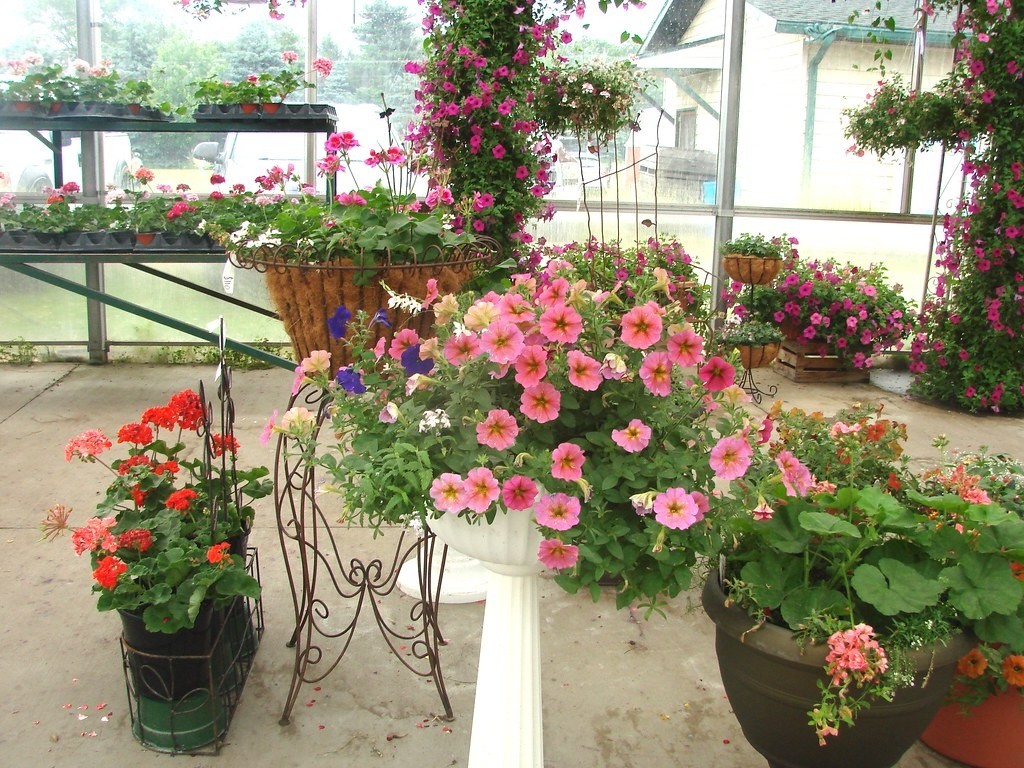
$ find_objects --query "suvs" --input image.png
[0,129,131,193]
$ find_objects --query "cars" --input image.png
[191,104,421,198]
[557,138,604,180]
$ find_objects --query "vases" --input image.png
[134,231,156,246]
[414,452,581,575]
[187,230,209,245]
[83,100,98,114]
[218,103,235,115]
[127,103,141,115]
[263,102,283,115]
[111,229,133,245]
[261,256,473,384]
[50,100,62,113]
[62,231,82,245]
[16,100,29,112]
[65,100,80,112]
[920,627,1024,768]
[198,104,212,115]
[86,231,107,244]
[241,102,260,114]
[775,311,811,341]
[7,228,28,243]
[113,575,221,705]
[160,231,182,246]
[719,339,784,370]
[396,532,501,606]
[36,231,53,245]
[703,560,985,768]
[180,514,253,619]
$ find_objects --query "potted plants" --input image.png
[719,232,783,284]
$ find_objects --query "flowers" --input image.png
[0,0,1024,739]
